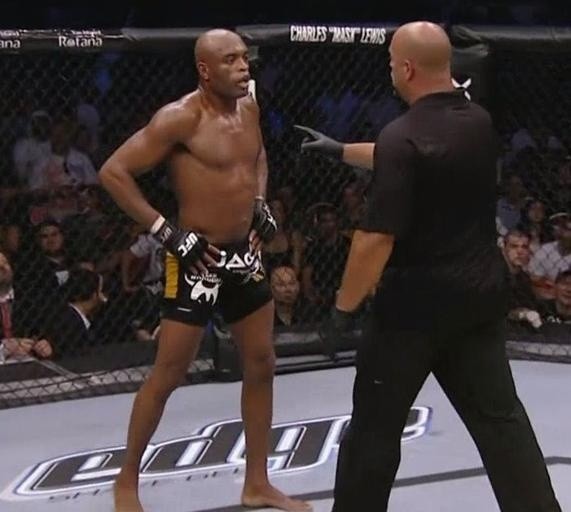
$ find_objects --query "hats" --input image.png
[30,109,53,125]
[73,104,116,144]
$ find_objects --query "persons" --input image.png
[0,39,571,376]
[291,17,564,512]
[94,25,317,512]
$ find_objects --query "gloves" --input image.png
[318,305,356,354]
[292,124,343,163]
